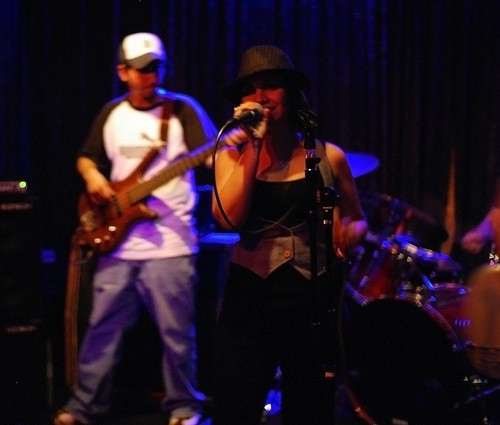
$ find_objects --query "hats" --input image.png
[118,32,166,69]
[222,45,310,102]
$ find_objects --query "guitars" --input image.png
[79,127,228,252]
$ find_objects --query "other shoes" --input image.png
[54,410,77,425]
[169,415,200,425]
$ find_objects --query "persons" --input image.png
[212,46,368,425]
[54,31,214,425]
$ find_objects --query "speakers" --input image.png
[0,196,55,425]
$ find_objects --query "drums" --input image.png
[344,231,418,308]
[345,282,469,425]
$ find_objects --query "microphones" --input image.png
[226,104,263,127]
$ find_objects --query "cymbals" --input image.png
[363,232,463,273]
[344,148,382,177]
[362,190,449,248]
